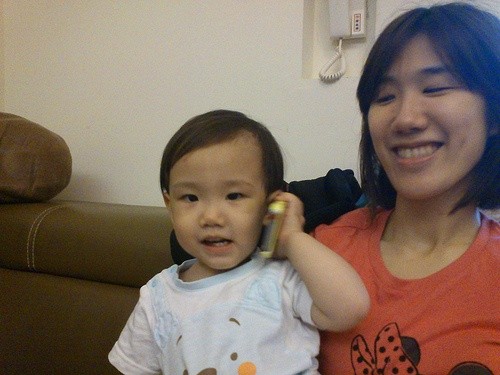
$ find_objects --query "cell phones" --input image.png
[259,200,286,258]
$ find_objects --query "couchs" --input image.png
[0,112,173,375]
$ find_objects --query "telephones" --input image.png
[328,0,366,41]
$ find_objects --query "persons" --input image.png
[308,2,500,375]
[107,109,370,375]
